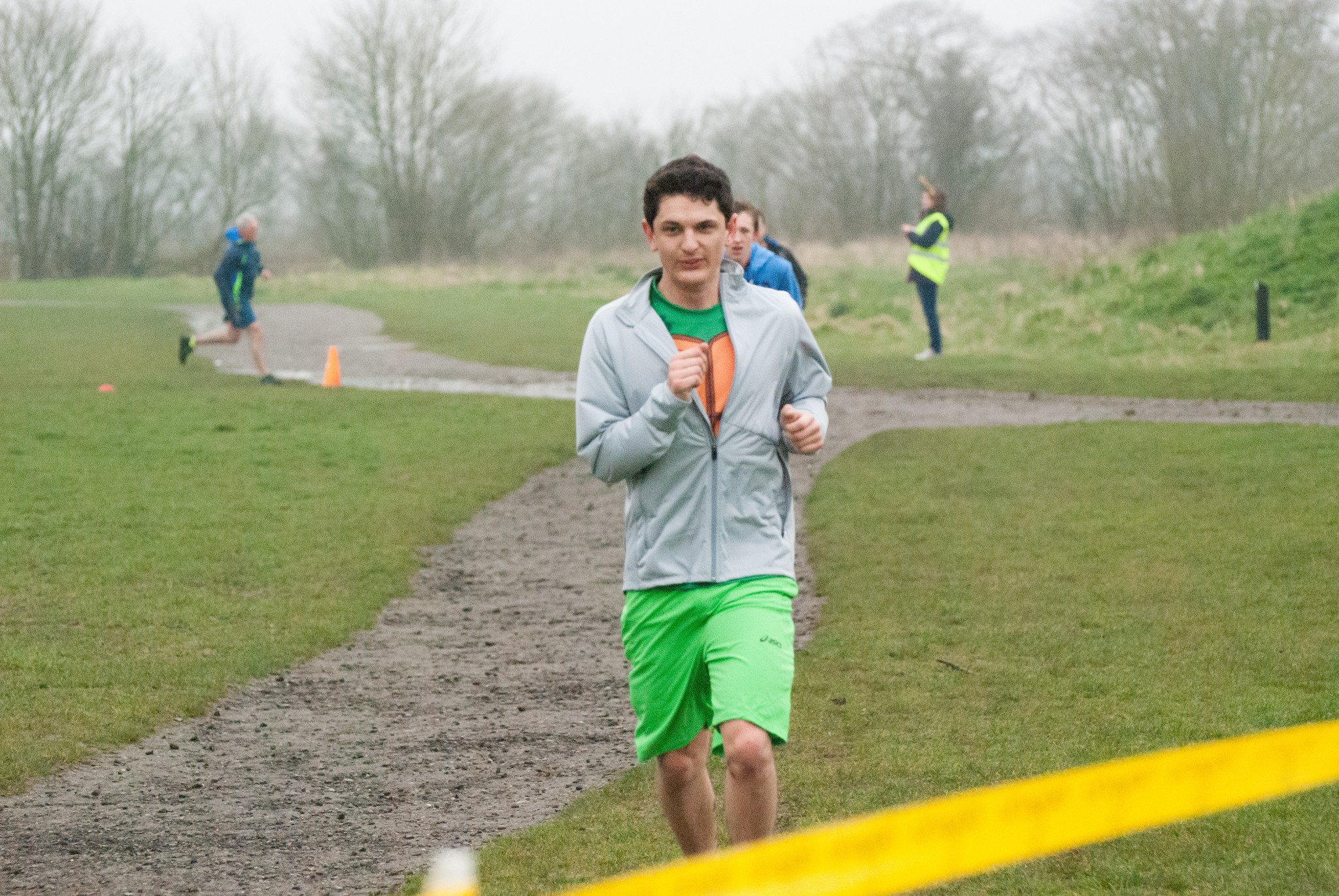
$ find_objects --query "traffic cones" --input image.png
[322,344,341,387]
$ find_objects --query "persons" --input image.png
[576,155,834,855]
[180,213,283,387]
[725,200,808,313]
[902,188,955,362]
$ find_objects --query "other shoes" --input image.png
[261,376,283,385]
[915,350,942,362]
[179,336,192,360]
